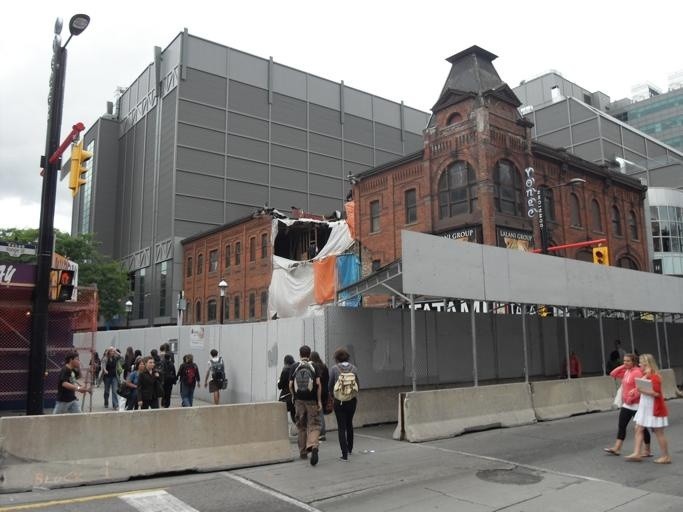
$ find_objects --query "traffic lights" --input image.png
[67,142,93,199]
[50,269,74,301]
[593,247,609,267]
[539,307,552,318]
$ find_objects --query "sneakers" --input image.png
[339,457,348,461]
[311,445,318,466]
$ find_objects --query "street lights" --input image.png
[25,11,90,417]
[537,176,586,254]
[125,299,134,328]
[215,278,229,325]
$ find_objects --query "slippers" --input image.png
[604,448,672,463]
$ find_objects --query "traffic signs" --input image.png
[0,241,37,256]
[51,252,79,272]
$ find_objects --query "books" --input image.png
[632,377,652,392]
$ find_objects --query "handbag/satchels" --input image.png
[117,382,131,398]
[156,382,165,398]
[614,385,624,408]
[326,391,332,415]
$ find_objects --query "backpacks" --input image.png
[212,362,226,383]
[333,364,358,401]
[185,366,196,386]
[293,361,315,394]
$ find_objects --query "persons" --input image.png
[602,353,655,459]
[275,353,297,425]
[609,338,627,366]
[327,347,362,462]
[606,350,624,376]
[287,344,323,466]
[569,351,582,378]
[630,348,641,363]
[49,340,228,416]
[308,351,329,442]
[624,354,671,465]
